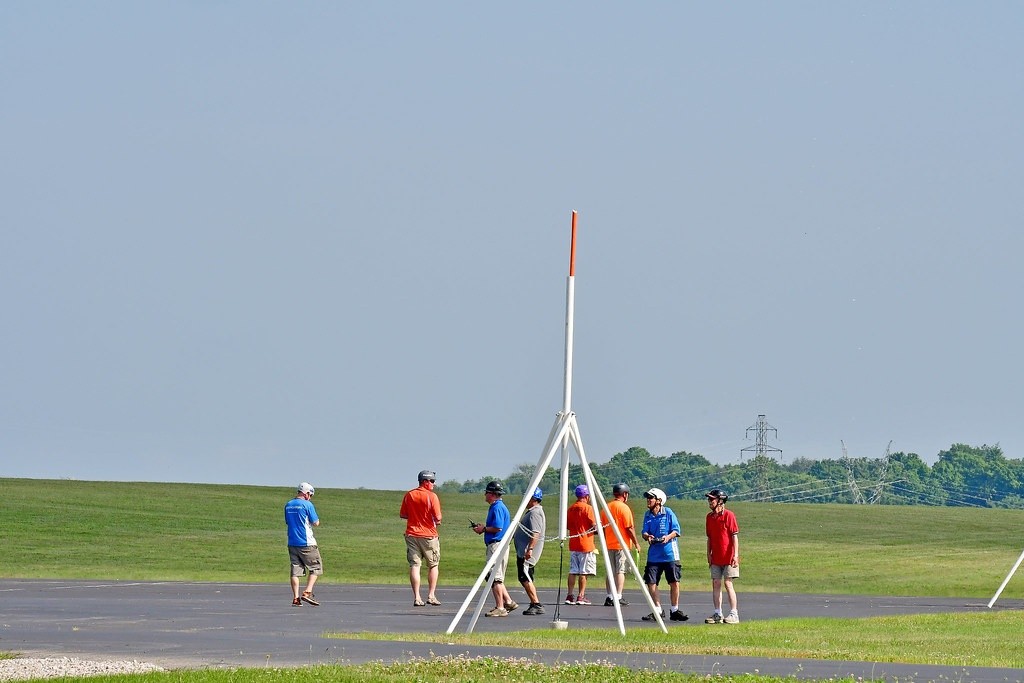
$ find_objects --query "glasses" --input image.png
[707,497,716,502]
[485,492,490,495]
[429,479,435,483]
[646,495,653,500]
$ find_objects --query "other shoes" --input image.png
[301,591,320,605]
[292,597,304,607]
[427,597,441,605]
[414,599,425,606]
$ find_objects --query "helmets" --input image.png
[532,487,543,501]
[418,470,436,480]
[705,489,728,504]
[575,485,589,498]
[297,482,314,496]
[612,483,629,498]
[484,481,506,495]
[644,488,666,507]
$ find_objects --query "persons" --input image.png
[513,487,546,615]
[400,470,442,606]
[473,481,519,617]
[705,489,740,624]
[565,485,598,605]
[600,483,641,606]
[641,487,689,622]
[284,483,323,607]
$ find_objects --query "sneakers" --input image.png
[670,609,689,621]
[485,607,508,617]
[576,595,592,605]
[522,601,545,615]
[723,611,739,623]
[641,610,665,622]
[705,613,724,624]
[504,600,519,613]
[604,596,629,605]
[565,594,576,605]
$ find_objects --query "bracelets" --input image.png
[482,527,486,532]
[528,547,533,550]
